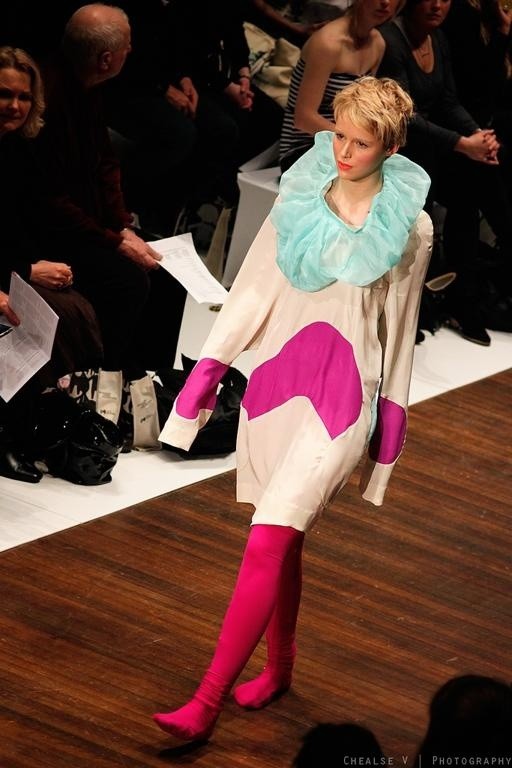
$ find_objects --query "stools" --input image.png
[220,167,281,289]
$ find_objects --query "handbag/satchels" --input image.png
[44,411,124,486]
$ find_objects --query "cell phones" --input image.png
[0,323,13,338]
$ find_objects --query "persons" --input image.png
[152,76,435,742]
[0,0,512,484]
[291,674,512,768]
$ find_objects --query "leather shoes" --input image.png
[0,445,43,483]
[464,322,490,346]
